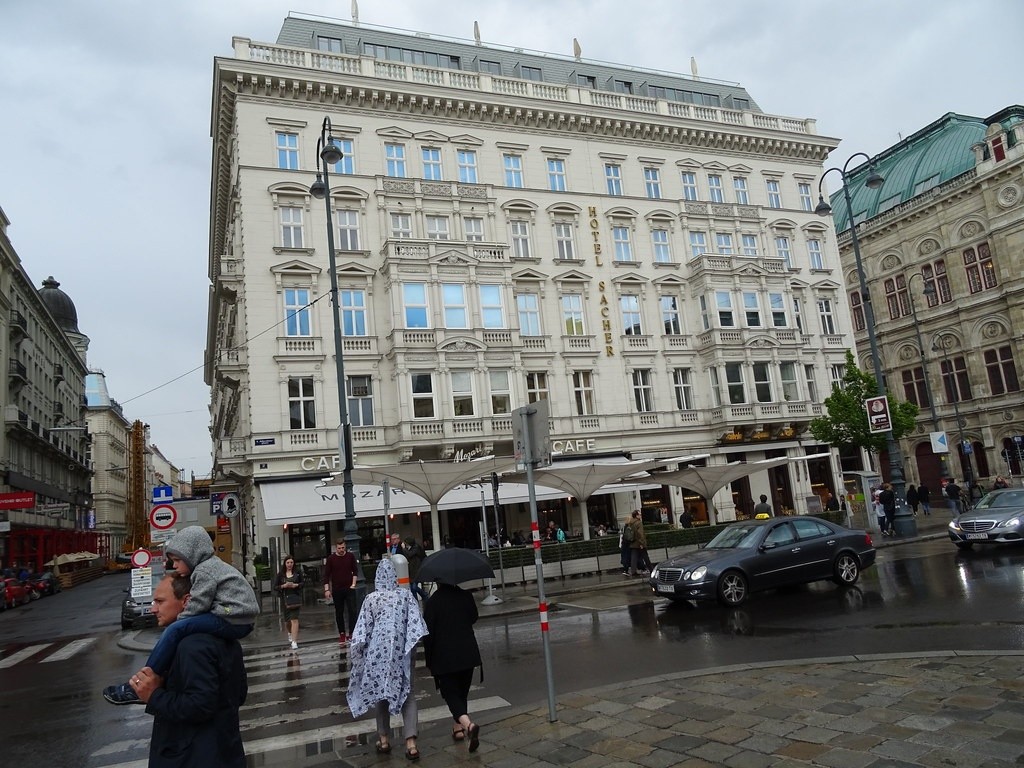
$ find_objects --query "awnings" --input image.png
[260,456,662,527]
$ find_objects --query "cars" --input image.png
[19,572,62,595]
[0,577,41,608]
[0,584,8,612]
[649,514,876,608]
[948,488,1024,548]
[120,570,179,628]
[931,333,976,504]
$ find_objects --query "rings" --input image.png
[136,679,139,685]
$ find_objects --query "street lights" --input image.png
[908,273,949,481]
[815,152,923,538]
[310,115,362,563]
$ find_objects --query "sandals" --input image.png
[405,746,419,760]
[451,728,465,741]
[376,741,392,752]
[467,723,480,752]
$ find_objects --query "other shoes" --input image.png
[632,573,640,577]
[288,633,292,641]
[340,632,346,642]
[291,642,298,650]
[884,530,890,536]
[349,634,352,640]
[622,571,630,577]
[642,570,649,574]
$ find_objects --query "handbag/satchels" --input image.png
[285,594,303,609]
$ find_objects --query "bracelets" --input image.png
[325,590,329,592]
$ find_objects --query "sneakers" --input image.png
[103,681,147,705]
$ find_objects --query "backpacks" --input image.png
[624,520,639,542]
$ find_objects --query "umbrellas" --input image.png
[413,545,496,586]
[43,551,100,565]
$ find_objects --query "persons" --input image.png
[324,539,358,644]
[129,571,248,768]
[824,492,839,511]
[389,533,403,554]
[994,476,1009,490]
[907,485,918,516]
[680,507,694,528]
[839,494,847,517]
[755,495,773,518]
[918,482,930,515]
[401,536,427,604]
[626,510,653,578]
[103,526,260,705]
[487,521,566,548]
[590,520,610,537]
[423,582,484,753]
[621,516,650,576]
[968,478,984,508]
[945,478,963,518]
[872,483,896,536]
[423,533,449,550]
[273,556,304,650]
[346,558,429,759]
